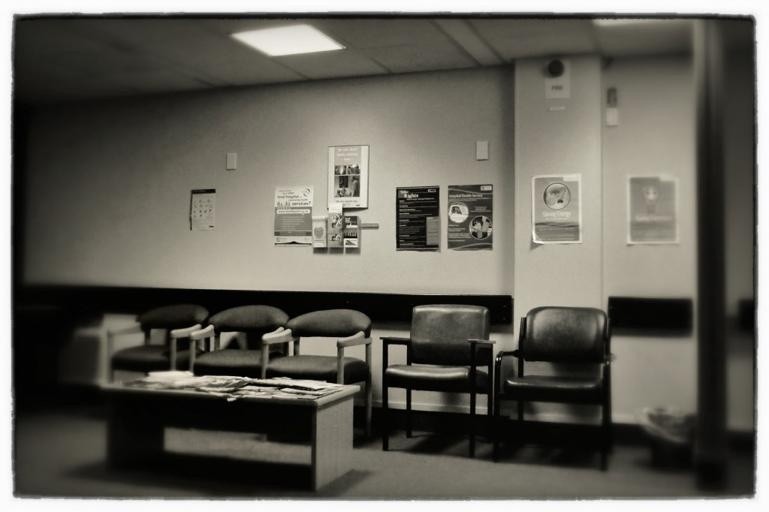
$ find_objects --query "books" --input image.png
[124,370,345,399]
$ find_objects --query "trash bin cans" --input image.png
[642,407,699,467]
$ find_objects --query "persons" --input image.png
[471,217,488,240]
[451,205,466,223]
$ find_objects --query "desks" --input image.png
[93,376,364,492]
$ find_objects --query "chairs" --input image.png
[494,306,618,469]
[100,304,209,370]
[262,310,373,424]
[379,303,499,459]
[190,306,288,379]
